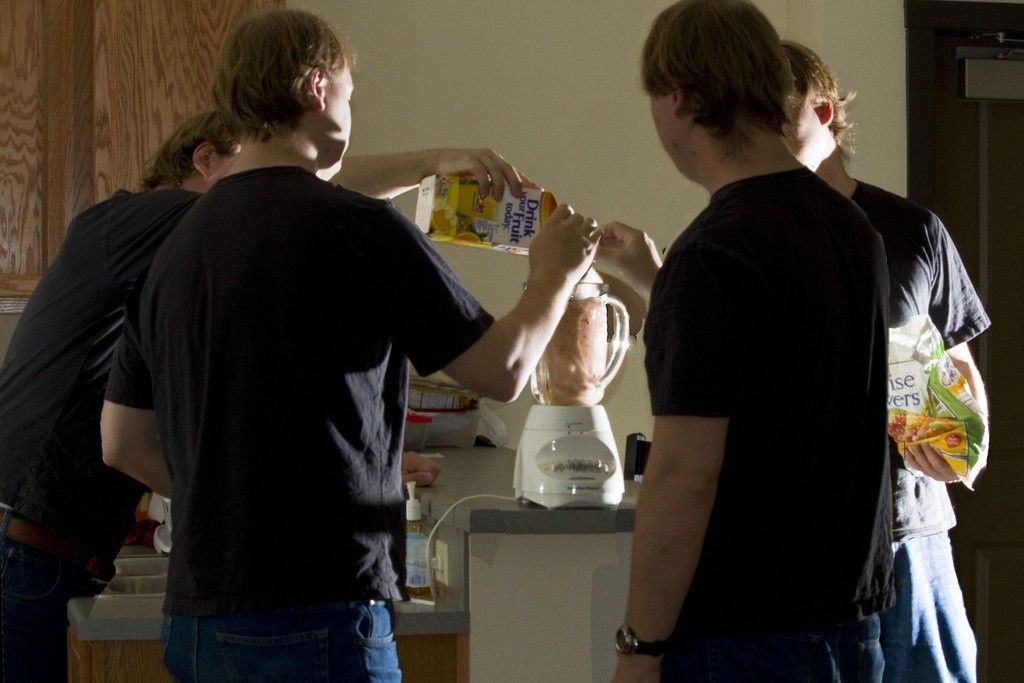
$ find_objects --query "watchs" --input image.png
[614,624,670,658]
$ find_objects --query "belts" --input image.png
[0,512,116,582]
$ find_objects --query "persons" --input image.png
[101,8,604,683]
[589,1,891,683]
[0,110,545,683]
[774,40,990,683]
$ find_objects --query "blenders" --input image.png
[511,279,633,510]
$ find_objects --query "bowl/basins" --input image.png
[403,416,432,451]
